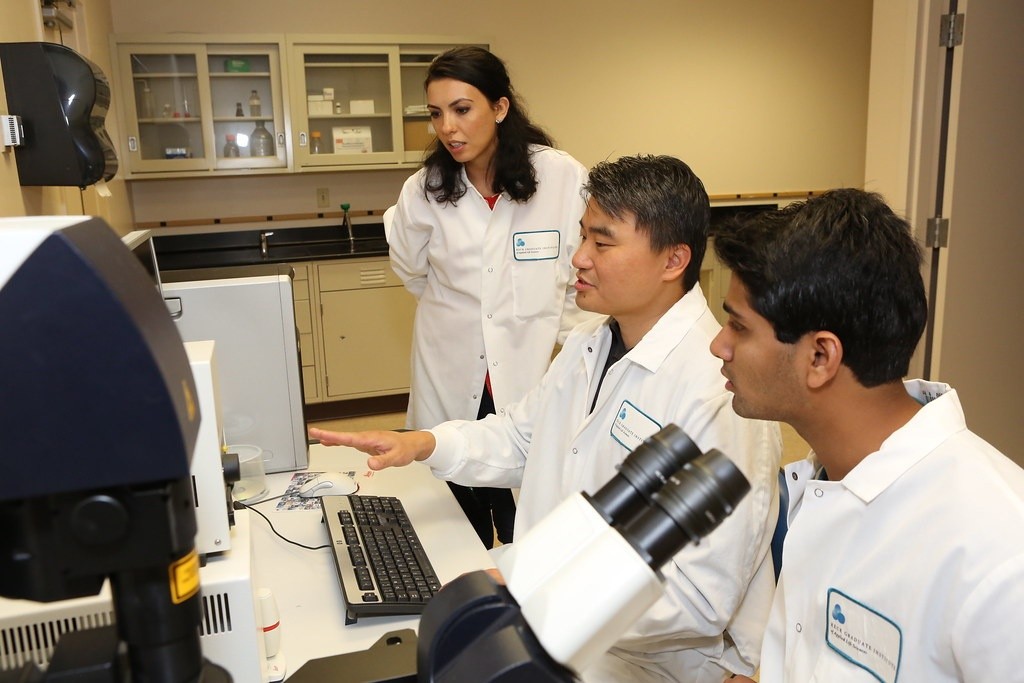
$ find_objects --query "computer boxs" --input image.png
[162,275,309,474]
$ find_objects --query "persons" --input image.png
[308,156,783,683]
[707,188,1024,683]
[384,47,599,551]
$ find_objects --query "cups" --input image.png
[226,445,267,501]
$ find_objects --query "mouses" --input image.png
[300,472,360,497]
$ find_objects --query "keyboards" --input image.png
[320,494,443,626]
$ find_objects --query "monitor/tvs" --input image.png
[121,229,165,304]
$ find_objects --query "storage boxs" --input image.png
[330,125,372,154]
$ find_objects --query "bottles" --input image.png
[249,90,261,116]
[236,103,243,116]
[336,103,341,114]
[223,135,240,158]
[140,88,156,118]
[309,131,326,154]
[249,121,274,156]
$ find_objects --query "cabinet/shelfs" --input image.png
[107,33,296,180]
[317,260,418,398]
[285,33,496,173]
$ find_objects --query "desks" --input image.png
[249,443,498,683]
